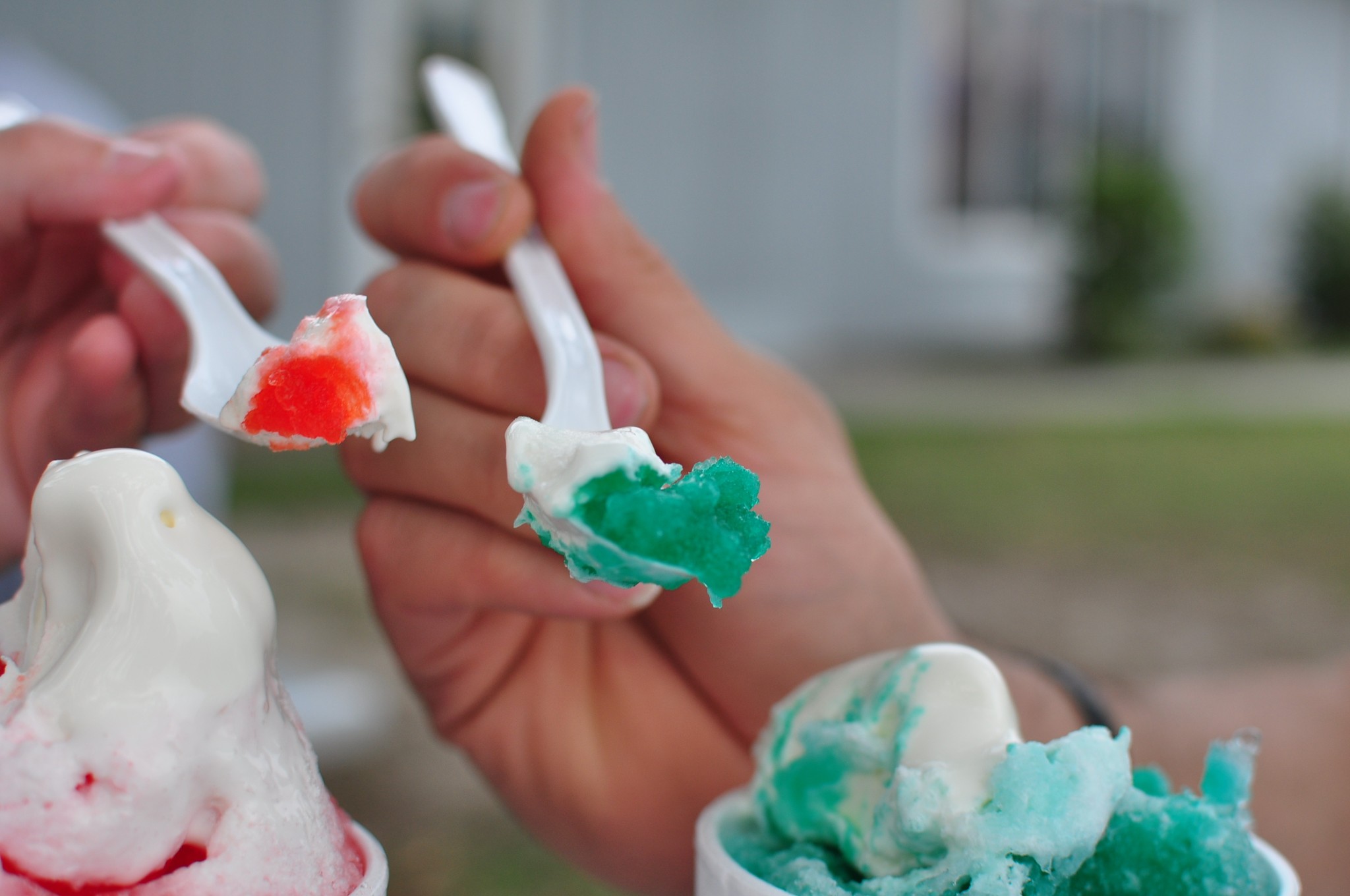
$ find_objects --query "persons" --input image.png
[296,78,1350,896]
[3,82,277,619]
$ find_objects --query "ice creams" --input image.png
[2,286,1305,895]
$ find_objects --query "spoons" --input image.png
[3,102,362,452]
[420,47,710,590]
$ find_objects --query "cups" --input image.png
[690,772,1302,894]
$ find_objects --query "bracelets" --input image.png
[1000,625,1126,757]
